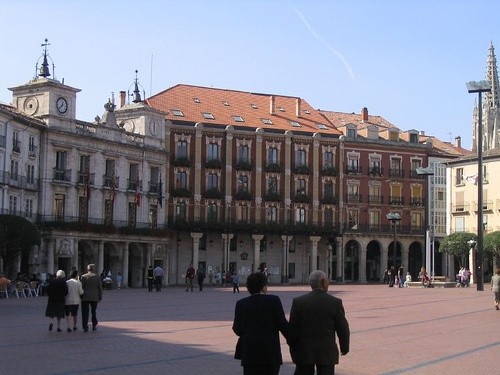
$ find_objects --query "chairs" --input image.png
[0,281,42,298]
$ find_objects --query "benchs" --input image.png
[405,282,458,287]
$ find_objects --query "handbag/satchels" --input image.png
[388,270,391,275]
[234,338,242,359]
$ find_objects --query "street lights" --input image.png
[387,212,403,284]
[416,167,435,285]
[464,80,492,292]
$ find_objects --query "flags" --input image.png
[86,169,162,208]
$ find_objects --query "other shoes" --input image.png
[73,326,77,331]
[185,289,188,291]
[84,329,88,332]
[496,305,499,310]
[191,290,193,291]
[67,327,72,332]
[57,328,62,332]
[92,327,97,331]
[49,323,53,331]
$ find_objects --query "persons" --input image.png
[80,264,103,332]
[233,273,240,293]
[64,270,85,332]
[46,270,68,332]
[153,265,164,292]
[491,268,500,310]
[232,273,289,375]
[457,267,470,288]
[287,270,350,375]
[15,272,51,296]
[185,264,195,292]
[384,264,412,288]
[67,267,76,279]
[257,268,268,277]
[100,269,123,289]
[208,269,232,287]
[197,264,206,291]
[145,266,156,292]
[421,267,430,288]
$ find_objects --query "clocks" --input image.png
[57,98,68,114]
